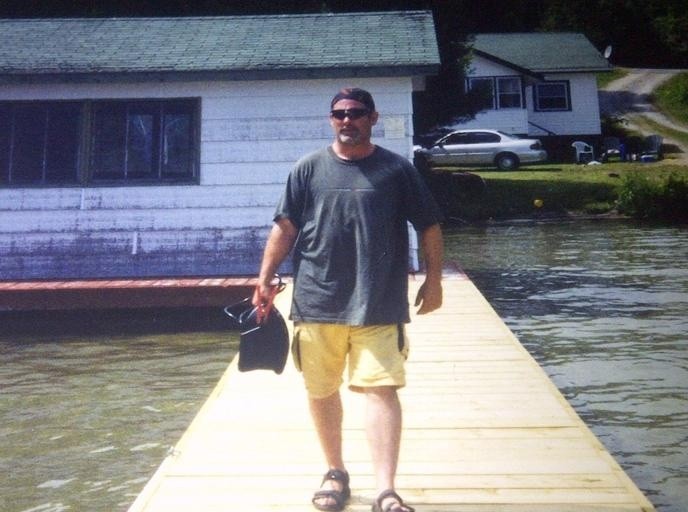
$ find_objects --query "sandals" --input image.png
[371,491,415,512]
[312,468,351,512]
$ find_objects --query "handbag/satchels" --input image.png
[224,296,289,375]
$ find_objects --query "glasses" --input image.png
[328,108,370,120]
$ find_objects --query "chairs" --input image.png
[571,134,664,164]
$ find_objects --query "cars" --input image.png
[417,129,548,170]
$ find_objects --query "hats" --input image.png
[330,88,375,111]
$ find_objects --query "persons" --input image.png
[255,88,441,512]
